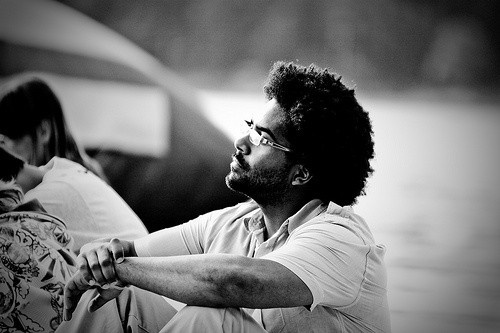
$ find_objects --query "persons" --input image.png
[1,189,75,333]
[1,80,104,182]
[60,58,390,333]
[0,148,148,257]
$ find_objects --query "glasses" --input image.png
[243,125,294,153]
